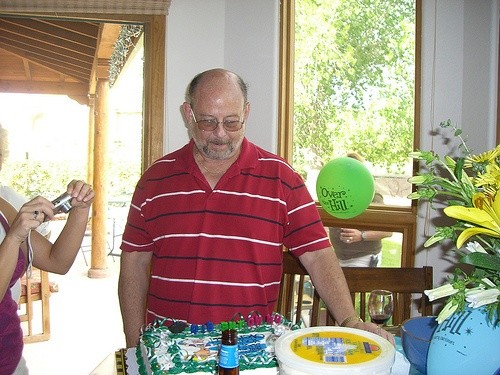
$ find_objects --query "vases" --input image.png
[427,303,500,375]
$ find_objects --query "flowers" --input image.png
[406,119,500,326]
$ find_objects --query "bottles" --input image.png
[217,328,240,374]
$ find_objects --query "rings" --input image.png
[34,211,39,218]
[350,237,352,239]
[347,240,349,241]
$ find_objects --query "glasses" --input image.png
[188,103,248,132]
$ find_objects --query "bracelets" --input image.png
[340,316,363,327]
[362,231,366,241]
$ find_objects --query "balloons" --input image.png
[426,305,500,375]
[316,157,375,219]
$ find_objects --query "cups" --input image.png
[368,290,394,328]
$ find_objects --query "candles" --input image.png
[191,313,281,333]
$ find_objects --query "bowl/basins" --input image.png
[399,312,444,374]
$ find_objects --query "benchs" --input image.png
[18,232,59,344]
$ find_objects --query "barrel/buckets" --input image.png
[273,326,395,375]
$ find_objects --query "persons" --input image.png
[0,126,95,375]
[328,152,393,267]
[118,68,396,348]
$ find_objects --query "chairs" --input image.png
[277,251,319,328]
[81,217,115,266]
[327,266,433,337]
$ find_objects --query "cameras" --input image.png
[42,192,74,223]
